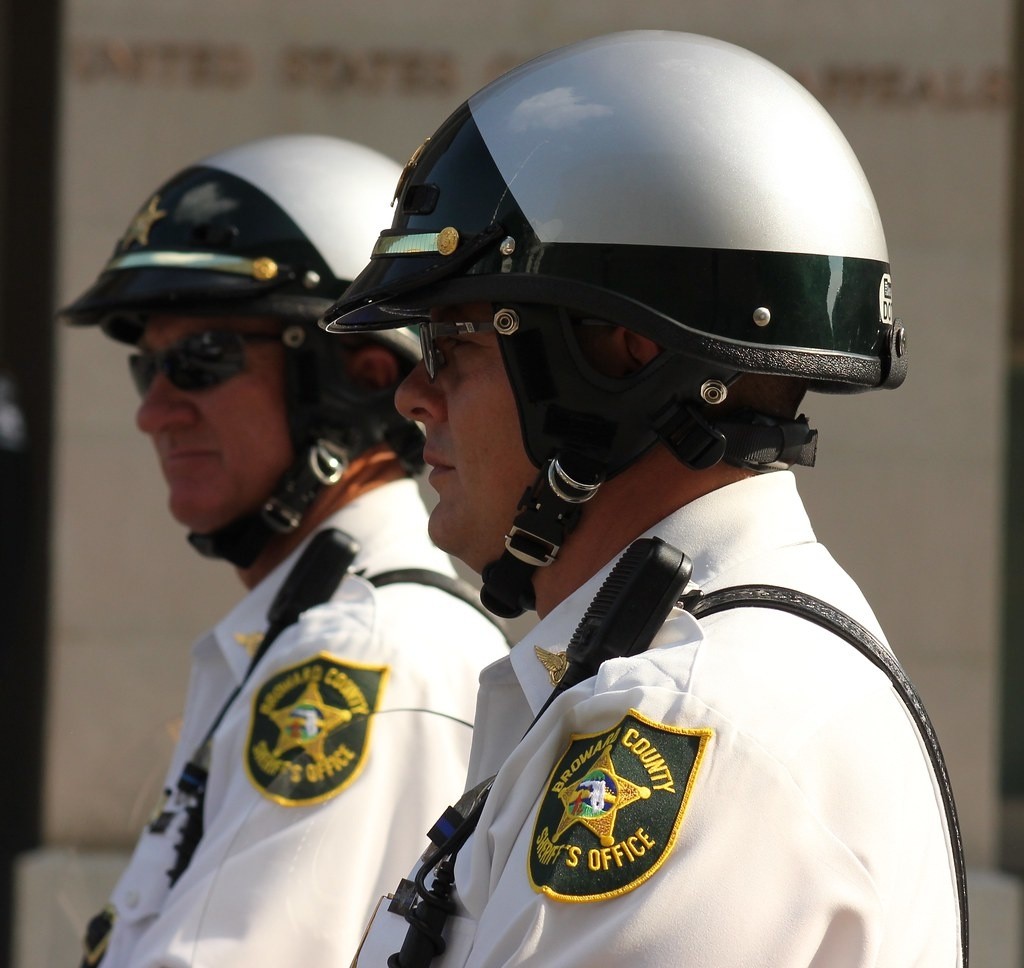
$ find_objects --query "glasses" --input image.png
[419,319,493,385]
[128,326,283,393]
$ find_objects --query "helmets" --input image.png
[317,29,906,396]
[56,134,426,361]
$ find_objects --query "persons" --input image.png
[319,31,972,968]
[60,137,512,968]
